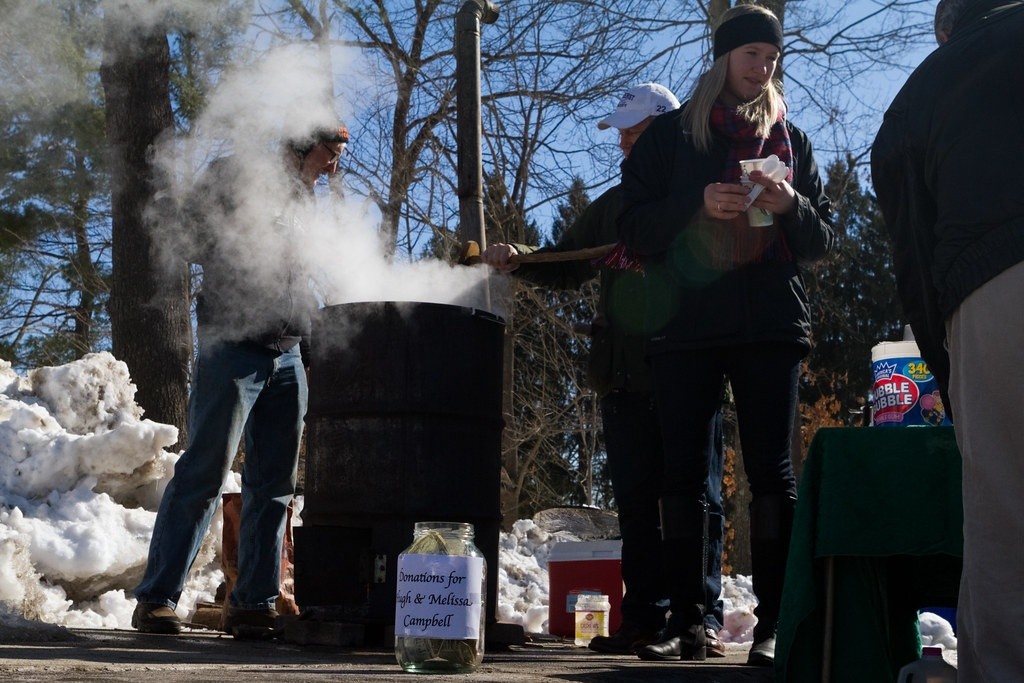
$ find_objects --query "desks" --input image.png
[766,426,965,683]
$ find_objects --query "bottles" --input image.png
[575,594,611,647]
[394,520,484,672]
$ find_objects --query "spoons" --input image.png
[743,155,789,209]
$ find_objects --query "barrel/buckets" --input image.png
[898,647,958,683]
[297,303,506,636]
[871,341,943,428]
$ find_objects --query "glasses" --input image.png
[322,142,340,165]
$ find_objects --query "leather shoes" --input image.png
[703,626,727,656]
[748,633,775,665]
[638,634,687,659]
[588,629,665,653]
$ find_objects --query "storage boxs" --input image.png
[547,539,624,638]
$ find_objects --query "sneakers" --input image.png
[131,603,181,635]
[231,623,278,643]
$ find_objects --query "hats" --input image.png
[291,102,349,160]
[598,83,683,131]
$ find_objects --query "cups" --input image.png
[739,159,774,226]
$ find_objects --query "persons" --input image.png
[130,93,352,647]
[479,80,730,660]
[869,1,1024,681]
[620,5,825,665]
